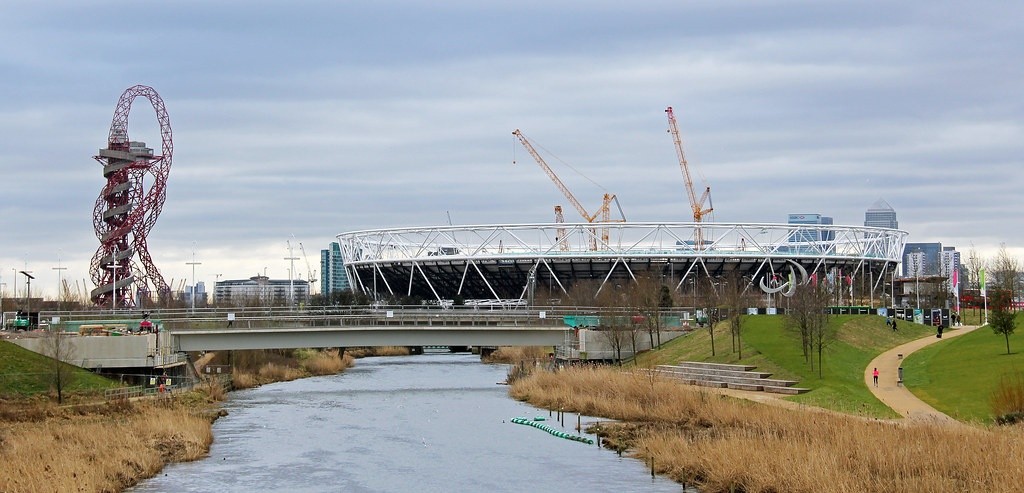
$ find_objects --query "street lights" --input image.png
[185,258,202,316]
[19,271,34,330]
[53,267,68,312]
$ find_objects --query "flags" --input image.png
[952,272,957,297]
[979,270,985,296]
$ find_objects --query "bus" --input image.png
[959,288,1014,311]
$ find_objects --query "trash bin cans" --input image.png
[898,367,903,378]
[898,353,903,359]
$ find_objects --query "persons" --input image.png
[227,321,232,327]
[957,315,961,323]
[892,320,897,331]
[886,317,892,326]
[873,367,879,387]
[938,325,943,334]
[951,314,956,323]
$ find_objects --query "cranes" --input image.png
[299,243,318,295]
[284,240,300,281]
[665,106,714,251]
[553,205,571,252]
[513,128,626,251]
